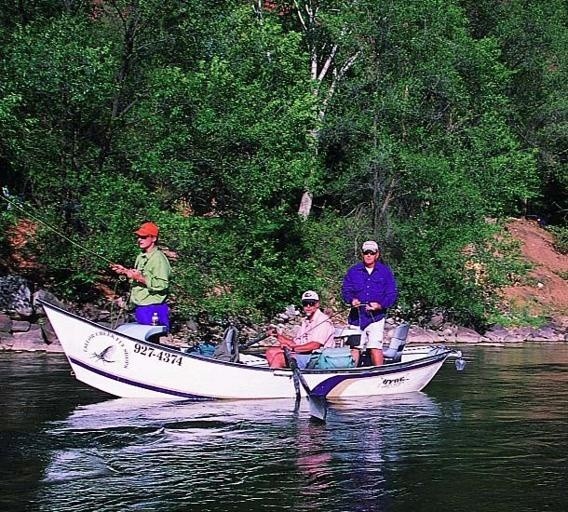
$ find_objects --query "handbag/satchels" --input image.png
[291,347,295,352]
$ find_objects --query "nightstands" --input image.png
[284,346,327,420]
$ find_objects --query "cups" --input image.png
[363,252,376,255]
[303,302,316,307]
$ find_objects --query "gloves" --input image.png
[39,298,465,396]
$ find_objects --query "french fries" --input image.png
[0,194,128,271]
[284,302,371,348]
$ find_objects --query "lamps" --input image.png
[383,319,413,364]
[344,334,361,368]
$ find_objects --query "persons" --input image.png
[107,222,170,338]
[341,240,398,366]
[266,289,336,370]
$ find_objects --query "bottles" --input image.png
[302,290,319,302]
[133,222,158,237]
[361,241,380,253]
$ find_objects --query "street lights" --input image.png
[311,346,355,368]
